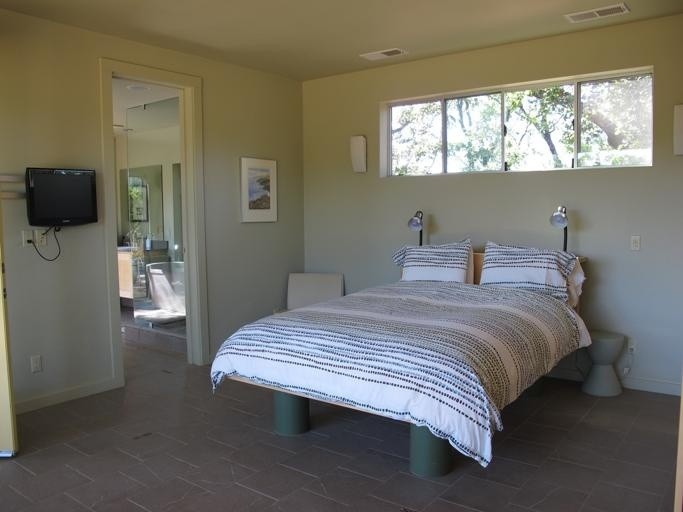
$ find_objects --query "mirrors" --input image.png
[120,164,164,241]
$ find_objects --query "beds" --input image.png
[211,251,588,477]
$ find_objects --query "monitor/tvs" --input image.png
[24,166,98,227]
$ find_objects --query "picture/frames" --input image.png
[130,183,149,224]
[240,155,279,224]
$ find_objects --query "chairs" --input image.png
[272,272,345,318]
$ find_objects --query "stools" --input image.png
[580,332,628,398]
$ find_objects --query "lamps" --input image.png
[407,206,426,251]
[548,205,574,251]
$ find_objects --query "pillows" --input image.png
[391,237,578,301]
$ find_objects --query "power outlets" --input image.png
[30,355,43,373]
[22,228,48,247]
[626,344,638,359]
